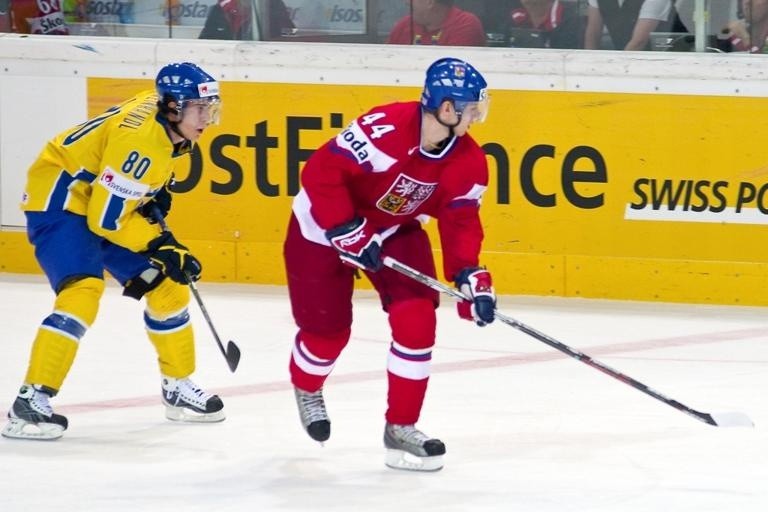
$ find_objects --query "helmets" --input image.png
[419,57,489,116]
[155,62,222,111]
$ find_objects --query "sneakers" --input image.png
[293,384,331,442]
[159,376,225,415]
[382,420,446,458]
[6,384,69,431]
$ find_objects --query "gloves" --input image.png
[137,186,172,225]
[140,230,203,286]
[323,212,385,275]
[454,264,498,327]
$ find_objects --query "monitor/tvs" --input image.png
[650,31,730,53]
[484,27,545,48]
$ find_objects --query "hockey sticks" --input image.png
[376,250,754,428]
[148,204,241,374]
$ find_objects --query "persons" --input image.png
[12,59,224,430]
[281,57,497,458]
[385,0,487,47]
[583,0,689,52]
[197,0,296,42]
[503,0,587,49]
